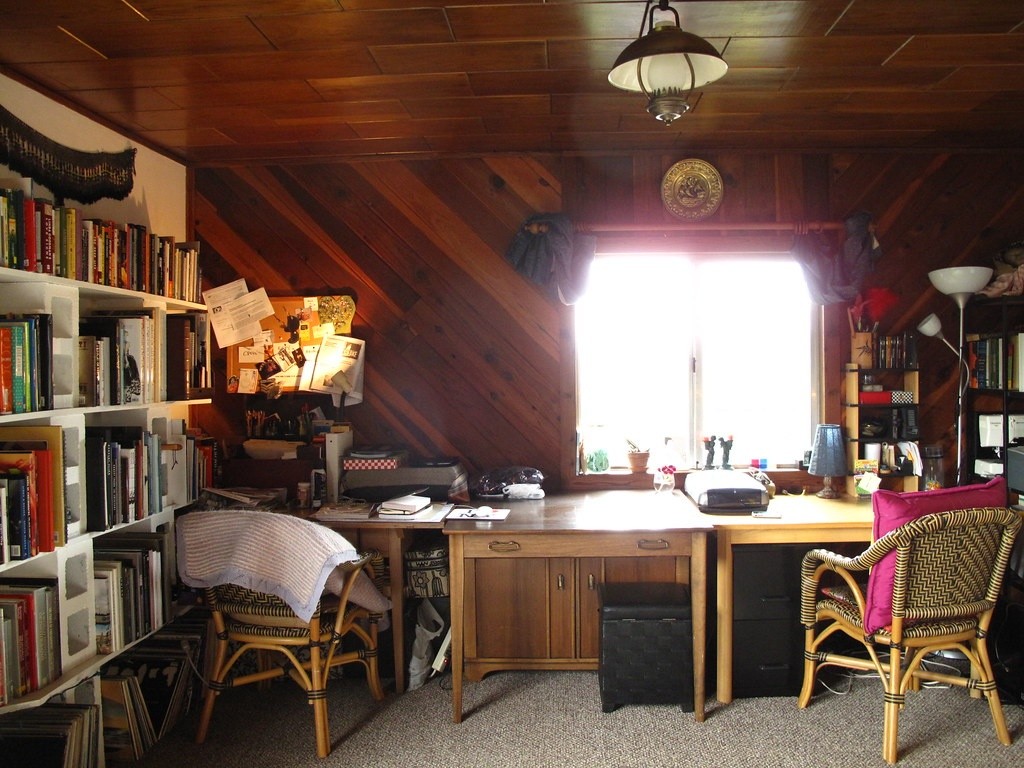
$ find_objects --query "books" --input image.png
[0,183,512,768]
[878,329,1024,440]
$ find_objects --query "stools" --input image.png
[593,579,696,718]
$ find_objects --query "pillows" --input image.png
[861,472,1008,638]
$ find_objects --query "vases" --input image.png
[654,472,676,493]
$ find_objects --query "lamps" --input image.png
[914,264,995,483]
[806,423,852,499]
[604,1,728,128]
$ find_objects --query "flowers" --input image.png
[720,434,735,469]
[658,464,677,476]
[703,435,718,470]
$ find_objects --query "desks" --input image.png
[686,488,874,705]
[442,489,714,723]
[204,493,452,699]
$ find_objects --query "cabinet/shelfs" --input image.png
[731,540,830,701]
[844,363,924,502]
[0,61,219,768]
[957,293,1024,498]
[461,534,692,686]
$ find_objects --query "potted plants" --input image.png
[626,438,651,473]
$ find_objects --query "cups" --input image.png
[865,443,880,466]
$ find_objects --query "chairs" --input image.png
[794,505,1023,768]
[173,509,384,760]
